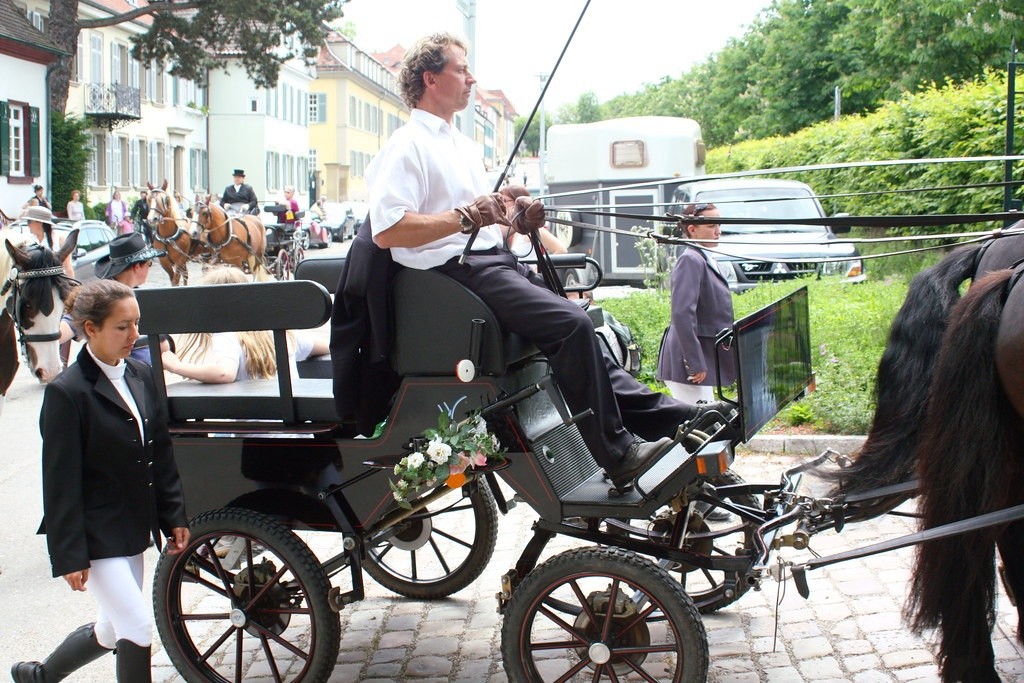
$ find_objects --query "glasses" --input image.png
[285,191,290,194]
[693,205,707,216]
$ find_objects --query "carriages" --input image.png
[145,179,305,286]
[134,218,1024,683]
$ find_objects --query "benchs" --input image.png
[132,253,606,428]
[262,204,305,240]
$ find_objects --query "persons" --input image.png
[60,232,169,368]
[105,190,127,227]
[163,266,330,558]
[11,279,190,683]
[130,191,153,248]
[116,212,132,233]
[23,185,52,209]
[657,203,737,520]
[174,193,184,210]
[497,185,567,272]
[241,437,346,512]
[220,168,258,215]
[567,283,593,305]
[363,33,738,486]
[314,195,327,220]
[20,205,75,368]
[273,185,300,233]
[67,190,84,220]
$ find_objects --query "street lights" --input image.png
[532,71,551,205]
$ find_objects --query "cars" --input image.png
[486,158,517,190]
[301,200,369,248]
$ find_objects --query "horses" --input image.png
[0,207,80,414]
[826,219,1024,683]
[143,180,265,287]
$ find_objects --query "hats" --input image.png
[94,232,168,280]
[22,206,54,226]
[232,169,246,177]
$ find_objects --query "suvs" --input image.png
[657,177,865,294]
[10,217,118,290]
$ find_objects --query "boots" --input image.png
[115,638,152,683]
[11,621,113,683]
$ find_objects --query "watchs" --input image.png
[459,213,472,231]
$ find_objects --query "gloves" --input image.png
[507,197,545,235]
[454,192,513,235]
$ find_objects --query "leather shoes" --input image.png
[604,437,673,486]
[695,500,731,520]
[681,396,738,454]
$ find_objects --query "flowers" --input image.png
[384,406,502,501]
